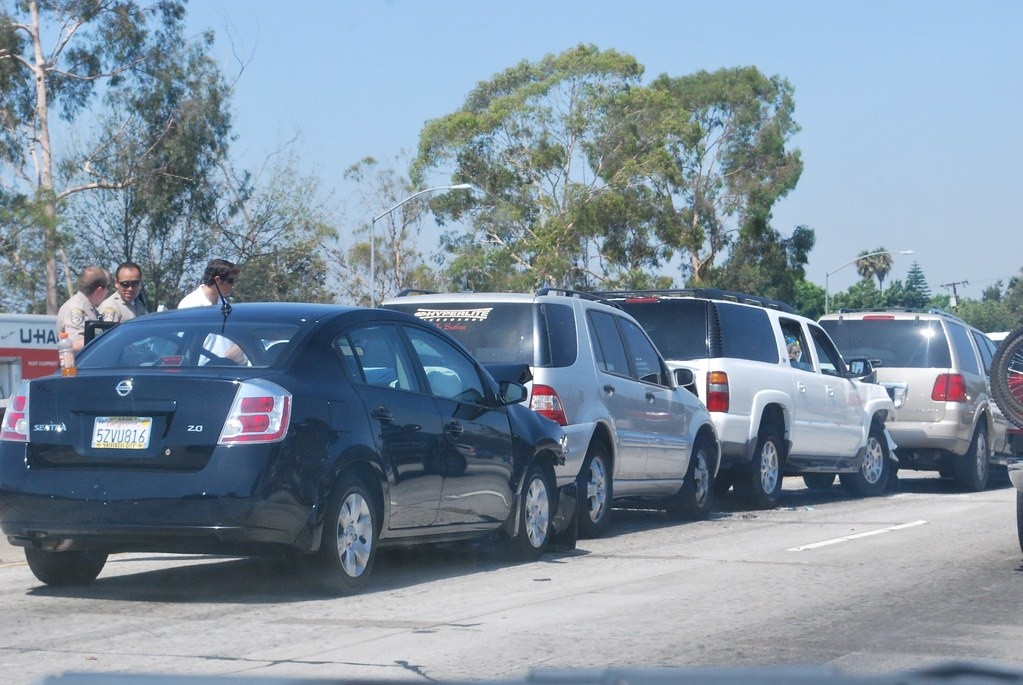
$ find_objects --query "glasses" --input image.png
[223,277,234,284]
[98,284,111,291]
[117,279,140,287]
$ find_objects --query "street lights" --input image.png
[369,183,473,308]
[824,250,915,314]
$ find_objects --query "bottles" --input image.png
[58,333,78,377]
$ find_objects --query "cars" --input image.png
[0,303,569,596]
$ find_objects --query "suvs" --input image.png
[358,287,722,550]
[816,306,1023,493]
[567,288,900,509]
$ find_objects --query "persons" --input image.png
[97,260,149,333]
[56,265,110,358]
[176,259,248,367]
